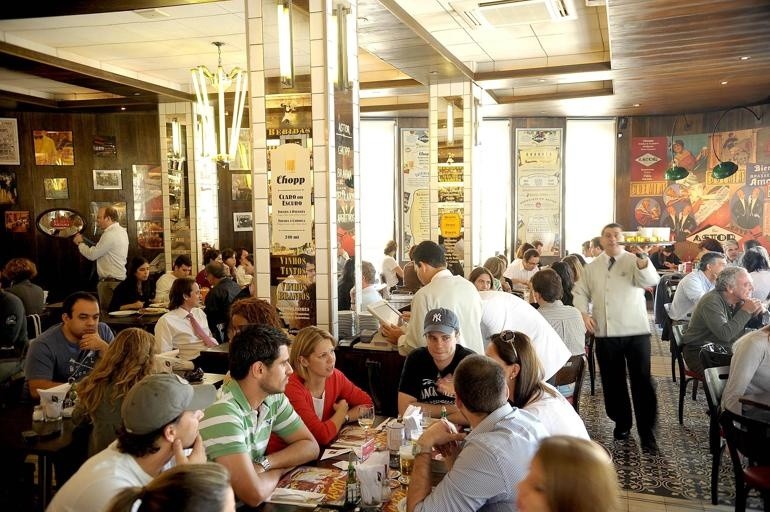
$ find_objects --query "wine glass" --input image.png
[357,405,374,445]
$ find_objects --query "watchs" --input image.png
[75,241,84,245]
[636,252,649,259]
[345,415,350,424]
[254,455,272,471]
[412,444,433,455]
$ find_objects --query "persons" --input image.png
[528,269,586,398]
[466,253,508,291]
[107,256,154,334]
[684,266,770,410]
[266,327,373,464]
[381,241,487,358]
[733,187,762,229]
[403,245,424,294]
[650,245,683,270]
[398,307,487,427]
[663,205,696,243]
[151,255,194,303]
[571,223,661,448]
[694,238,723,269]
[71,328,160,461]
[668,139,697,173]
[666,252,725,346]
[100,463,237,512]
[73,205,129,314]
[515,434,621,512]
[26,292,115,405]
[552,247,594,313]
[663,188,687,205]
[277,238,386,329]
[231,298,296,364]
[722,240,744,268]
[195,323,320,512]
[44,375,209,512]
[204,262,242,343]
[223,248,239,285]
[33,131,58,164]
[719,324,770,446]
[237,246,252,285]
[739,238,761,266]
[637,199,660,224]
[502,241,544,303]
[407,354,550,512]
[742,250,770,307]
[478,290,571,387]
[484,330,594,441]
[1,273,28,382]
[383,242,403,291]
[241,254,256,298]
[723,132,737,154]
[589,236,605,258]
[3,257,45,319]
[153,278,219,375]
[196,248,231,292]
[581,240,595,258]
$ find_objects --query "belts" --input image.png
[99,275,121,285]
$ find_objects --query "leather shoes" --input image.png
[613,428,631,441]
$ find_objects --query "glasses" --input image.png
[526,260,540,268]
[500,330,519,359]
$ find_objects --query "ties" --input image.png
[187,312,216,347]
[608,257,615,271]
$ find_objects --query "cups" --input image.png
[419,407,432,426]
[400,453,415,478]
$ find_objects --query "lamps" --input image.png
[664,111,689,181]
[277,0,294,90]
[447,153,455,165]
[711,105,759,180]
[447,100,454,145]
[191,41,247,167]
[172,118,181,159]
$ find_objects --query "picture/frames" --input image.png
[36,208,88,239]
[4,210,30,233]
[93,169,122,191]
[0,117,20,165]
[0,170,17,205]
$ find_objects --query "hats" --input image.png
[423,307,458,337]
[660,246,676,251]
[121,371,217,434]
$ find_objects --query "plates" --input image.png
[109,310,139,318]
[150,302,167,307]
[338,310,355,336]
[138,306,169,317]
[358,310,378,331]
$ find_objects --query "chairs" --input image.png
[702,365,731,506]
[718,409,770,512]
[665,279,678,352]
[25,312,48,340]
[670,323,712,424]
[664,303,689,383]
[554,355,585,414]
[585,332,596,396]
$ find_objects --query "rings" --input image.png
[85,341,88,346]
